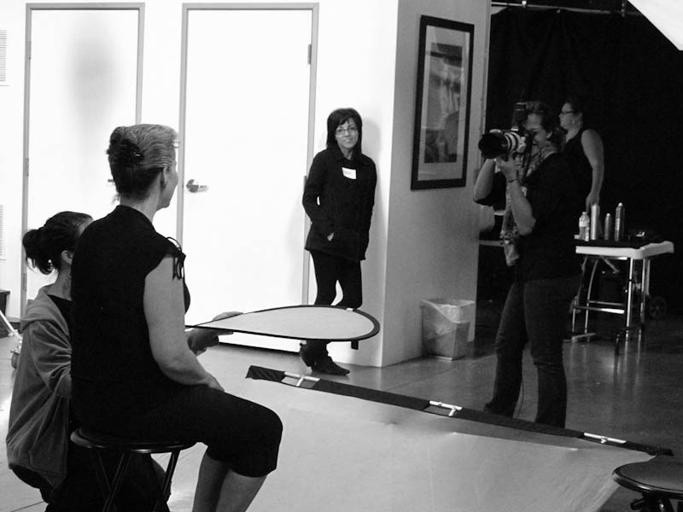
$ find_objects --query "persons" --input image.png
[551,100,603,212]
[300,108,377,376]
[69,125,282,511]
[3,212,172,511]
[473,100,577,429]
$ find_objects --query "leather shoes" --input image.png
[309,354,350,376]
[299,343,314,367]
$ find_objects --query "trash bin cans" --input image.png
[421,296,476,359]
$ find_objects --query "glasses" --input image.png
[334,127,358,136]
[559,111,581,114]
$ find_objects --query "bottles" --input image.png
[579,211,589,242]
[604,213,613,240]
[590,203,600,240]
[614,202,626,241]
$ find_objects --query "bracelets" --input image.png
[506,178,518,183]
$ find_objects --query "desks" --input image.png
[571,241,674,355]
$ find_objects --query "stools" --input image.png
[69,428,195,512]
[612,461,683,511]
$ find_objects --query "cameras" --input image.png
[478,103,533,160]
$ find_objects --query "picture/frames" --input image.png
[411,15,474,189]
